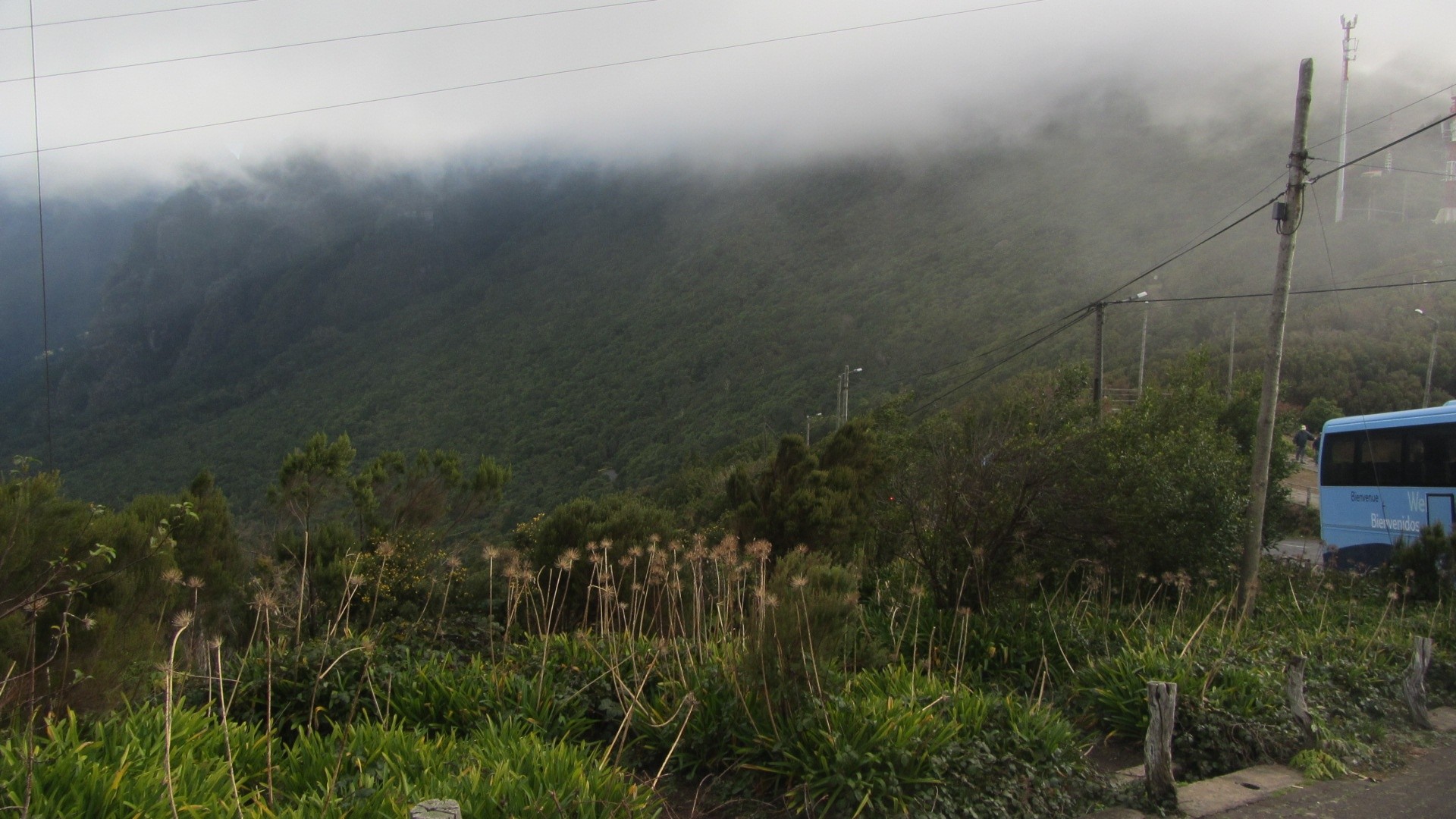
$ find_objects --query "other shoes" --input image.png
[1300,461,1306,464]
[1315,462,1318,464]
[1296,459,1300,461]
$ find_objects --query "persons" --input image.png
[1312,432,1321,465]
[1293,425,1316,464]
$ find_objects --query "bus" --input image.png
[1318,401,1456,580]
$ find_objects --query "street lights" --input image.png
[806,413,823,450]
[837,368,863,430]
[1095,291,1149,428]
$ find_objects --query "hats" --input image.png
[1319,432,1322,436]
[1301,425,1306,431]
